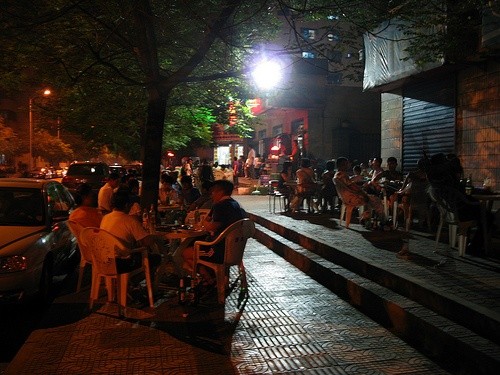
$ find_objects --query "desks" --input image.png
[471,192,500,215]
[145,223,209,301]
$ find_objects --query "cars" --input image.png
[0,176,82,311]
[0,160,143,187]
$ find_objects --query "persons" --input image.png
[0,161,35,178]
[99,191,162,300]
[247,144,256,179]
[119,154,264,191]
[174,176,201,205]
[182,180,248,287]
[169,181,215,256]
[278,154,500,250]
[97,171,120,213]
[156,173,178,205]
[124,179,141,208]
[68,184,102,264]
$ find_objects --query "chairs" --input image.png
[268,179,477,256]
[65,208,253,319]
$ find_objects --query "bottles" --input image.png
[143,211,149,229]
[193,207,200,230]
[166,194,170,205]
[149,204,156,227]
[465,176,472,195]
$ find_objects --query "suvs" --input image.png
[62,159,113,206]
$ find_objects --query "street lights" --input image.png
[29,87,52,169]
